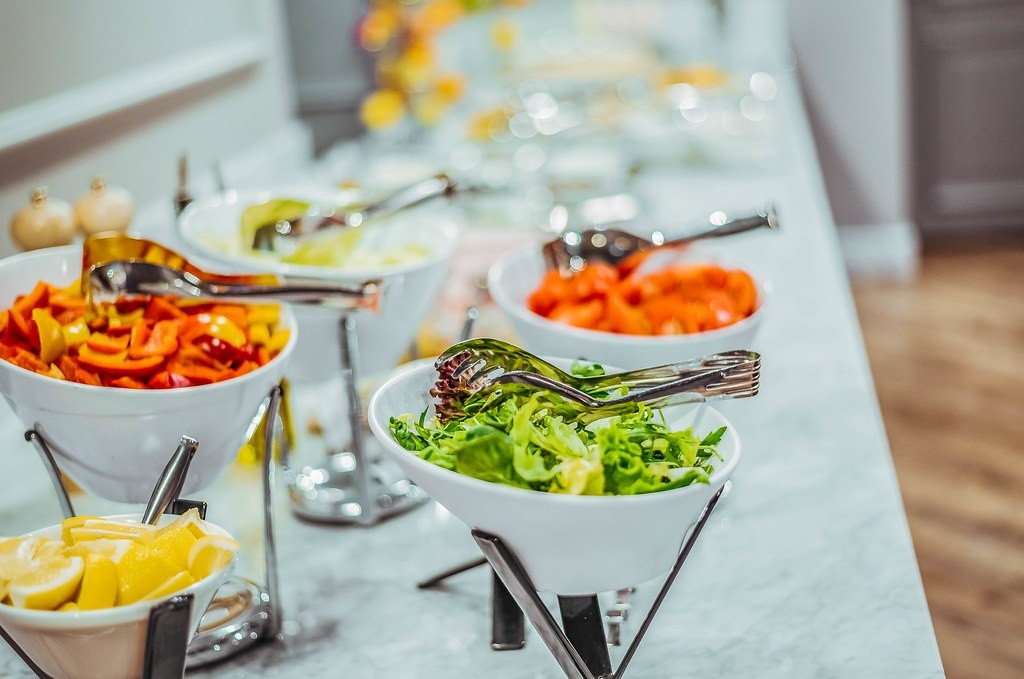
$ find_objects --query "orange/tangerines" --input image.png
[0,516,242,610]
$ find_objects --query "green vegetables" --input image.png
[389,336,729,496]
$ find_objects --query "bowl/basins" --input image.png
[1,246,297,504]
[1,513,237,679]
[369,357,739,592]
[492,241,771,370]
[180,182,445,385]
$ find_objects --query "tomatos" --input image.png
[527,249,758,337]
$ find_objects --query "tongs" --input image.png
[240,174,456,254]
[546,206,777,280]
[433,337,761,437]
[141,435,199,525]
[81,232,378,307]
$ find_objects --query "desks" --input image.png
[0,37,945,679]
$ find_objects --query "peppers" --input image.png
[0,277,292,389]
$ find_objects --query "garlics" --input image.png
[10,178,131,250]
